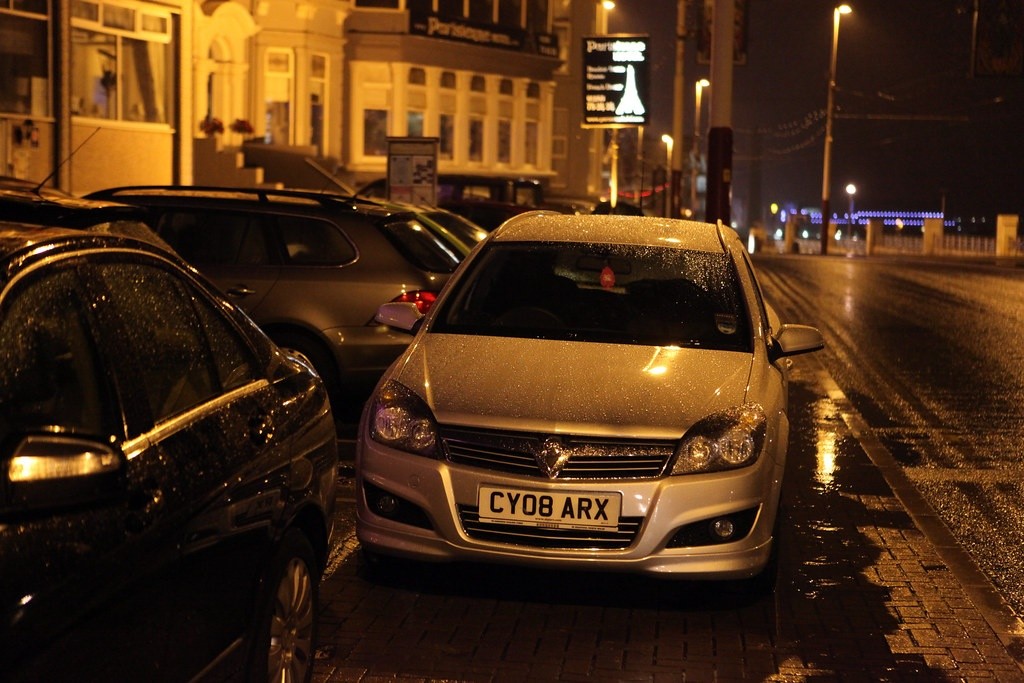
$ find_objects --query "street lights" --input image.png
[818,3,854,254]
[687,79,710,209]
[843,181,857,234]
[659,134,675,218]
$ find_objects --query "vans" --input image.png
[352,170,548,208]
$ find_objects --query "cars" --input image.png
[353,204,826,592]
[0,218,341,682]
[0,172,183,264]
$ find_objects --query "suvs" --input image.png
[80,182,472,397]
